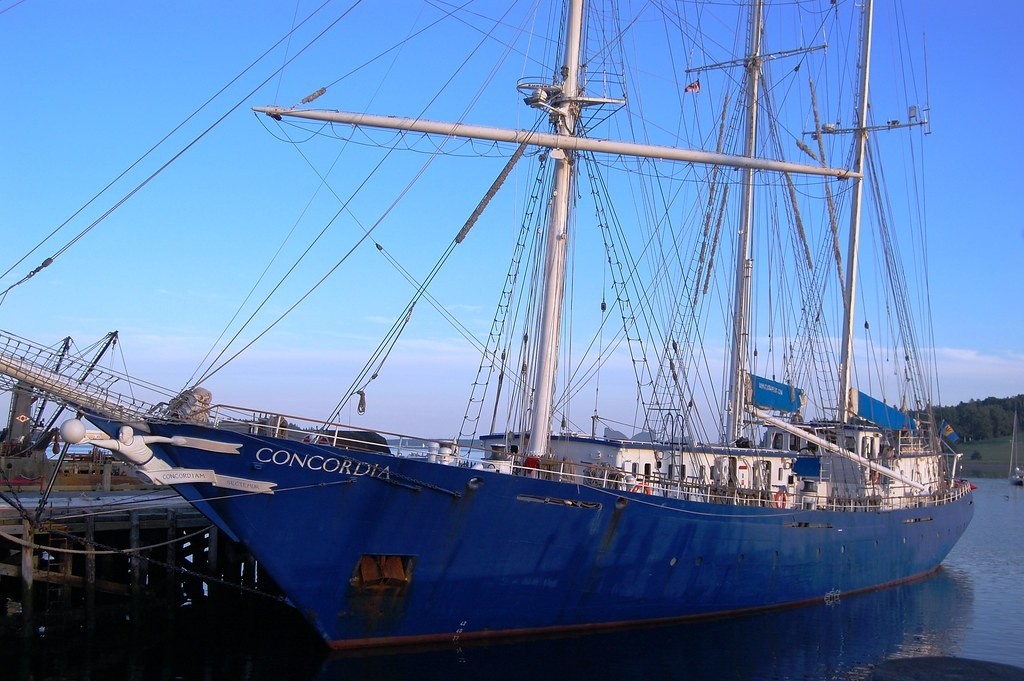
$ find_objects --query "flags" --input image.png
[685,79,700,93]
[938,421,960,444]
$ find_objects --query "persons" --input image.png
[883,445,895,458]
[76,426,187,473]
[900,425,908,443]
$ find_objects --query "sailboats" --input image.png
[0,1,978,657]
[1006,403,1024,486]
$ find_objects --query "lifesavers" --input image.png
[772,490,788,510]
[630,482,652,495]
